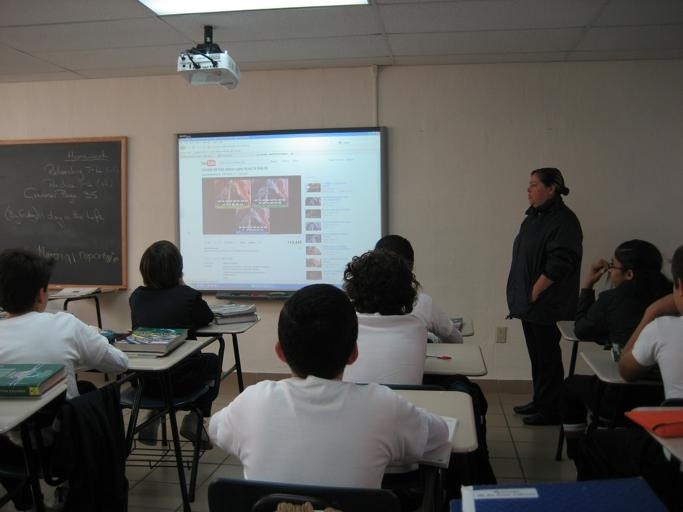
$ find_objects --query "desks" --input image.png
[48,289,103,331]
[634,406,683,470]
[579,349,661,383]
[392,388,480,454]
[422,341,487,376]
[427,312,477,339]
[0,384,70,512]
[193,316,259,393]
[556,319,605,461]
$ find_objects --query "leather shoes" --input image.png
[522,411,560,426]
[513,400,536,414]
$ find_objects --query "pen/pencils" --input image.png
[426,355,452,359]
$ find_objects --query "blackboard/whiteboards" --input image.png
[0,136,128,291]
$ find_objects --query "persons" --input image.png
[617,244,683,404]
[375,234,463,346]
[208,283,450,490]
[0,248,130,512]
[129,240,222,450]
[558,239,674,462]
[505,168,583,425]
[335,249,429,386]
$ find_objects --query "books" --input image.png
[48,287,119,299]
[423,414,669,511]
[430,318,463,343]
[99,302,258,359]
[1,363,69,399]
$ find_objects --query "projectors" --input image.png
[176,52,240,91]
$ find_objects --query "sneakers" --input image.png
[137,419,159,445]
[179,413,212,449]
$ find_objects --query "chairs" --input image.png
[208,478,399,512]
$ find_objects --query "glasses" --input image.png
[607,261,628,273]
